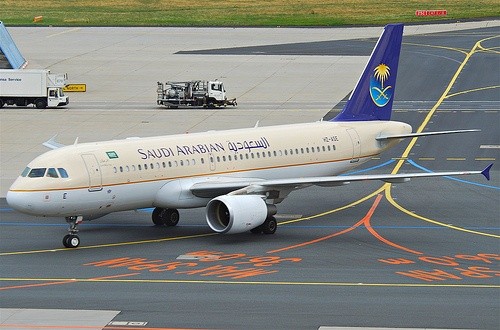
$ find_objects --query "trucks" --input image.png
[0,68,70,109]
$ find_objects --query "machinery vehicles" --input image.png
[156,79,237,110]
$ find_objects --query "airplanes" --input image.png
[5,22,494,249]
